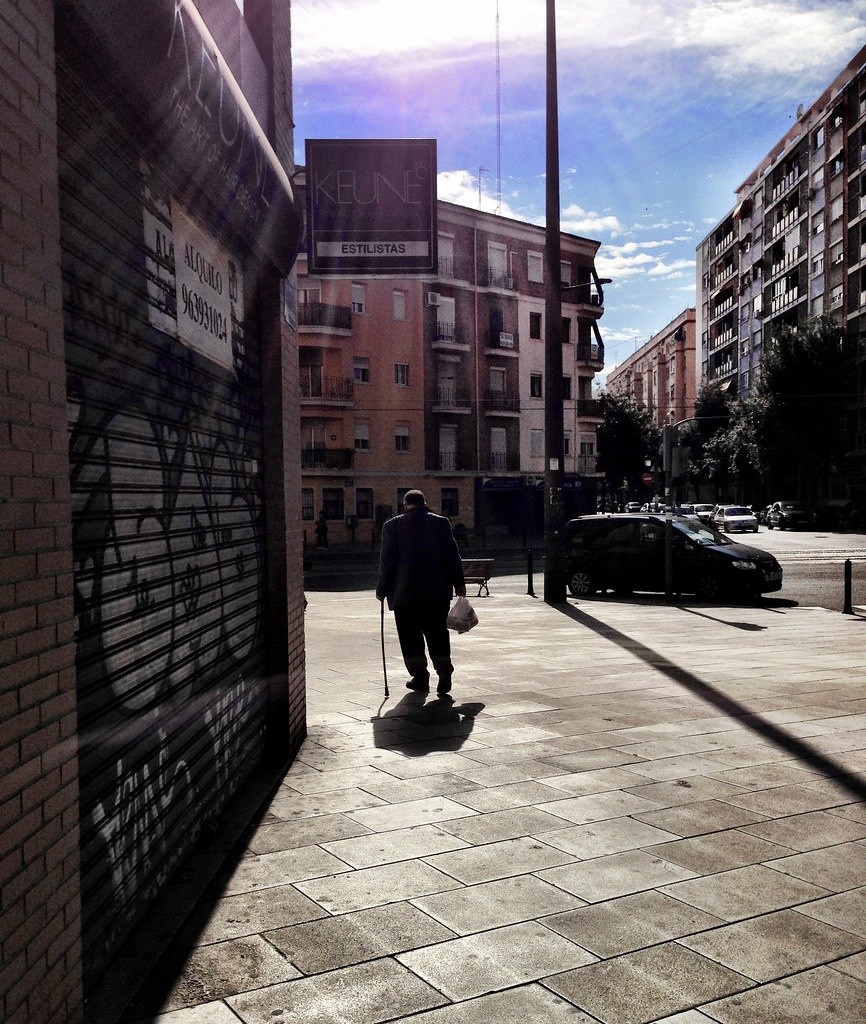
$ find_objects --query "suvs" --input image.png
[557,513,782,606]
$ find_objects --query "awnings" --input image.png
[732,199,753,219]
[668,327,684,341]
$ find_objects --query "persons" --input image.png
[316,511,329,551]
[375,490,466,694]
[454,522,468,548]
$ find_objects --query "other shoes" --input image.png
[405,678,430,692]
[437,678,451,694]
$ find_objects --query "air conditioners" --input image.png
[500,278,513,290]
[424,291,442,308]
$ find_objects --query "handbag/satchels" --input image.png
[458,598,479,635]
[447,596,469,632]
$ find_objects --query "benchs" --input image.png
[460,556,497,598]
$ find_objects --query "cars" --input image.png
[766,500,816,531]
[624,501,759,536]
